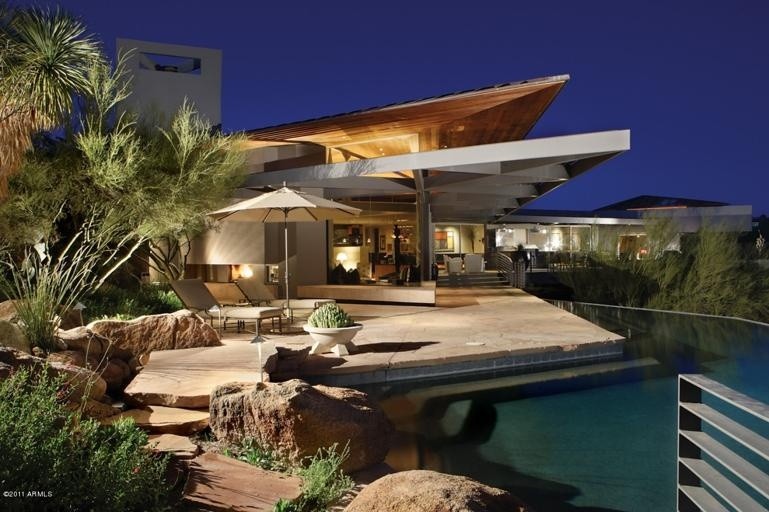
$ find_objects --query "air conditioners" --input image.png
[264,264,279,283]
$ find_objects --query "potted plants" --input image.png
[303,301,364,357]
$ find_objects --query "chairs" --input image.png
[168,277,336,337]
[443,253,485,273]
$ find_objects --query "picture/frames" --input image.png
[380,235,385,249]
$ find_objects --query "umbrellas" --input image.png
[205,179,363,328]
